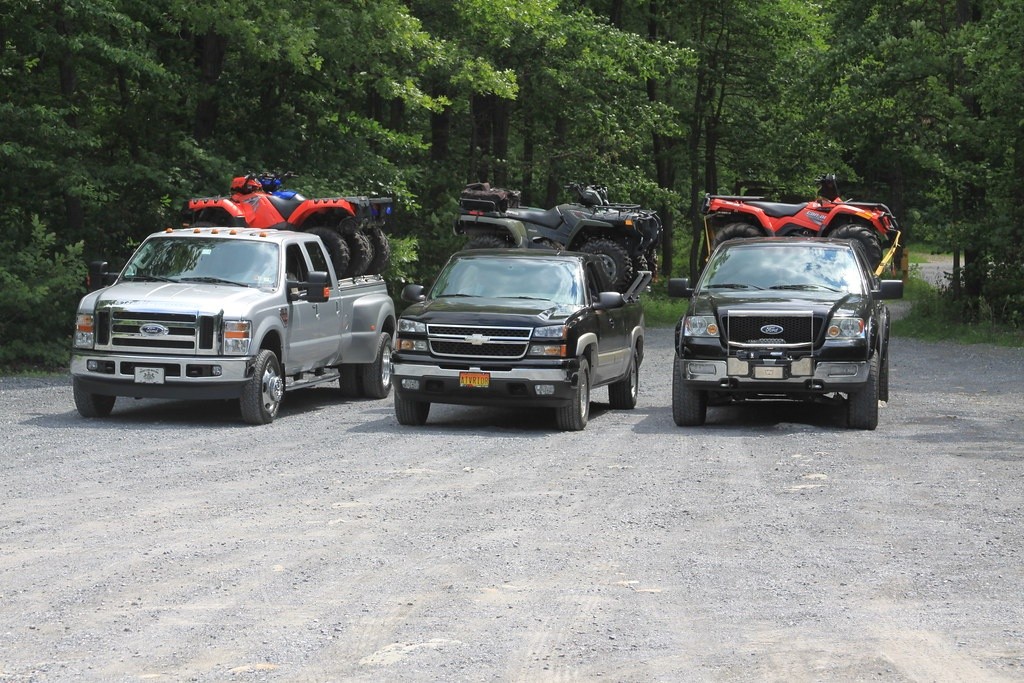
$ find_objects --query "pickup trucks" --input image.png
[669,235,904,430]
[386,247,646,432]
[71,224,397,425]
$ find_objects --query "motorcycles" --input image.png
[175,172,399,276]
[451,182,665,301]
[693,173,908,299]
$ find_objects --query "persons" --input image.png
[266,252,297,281]
